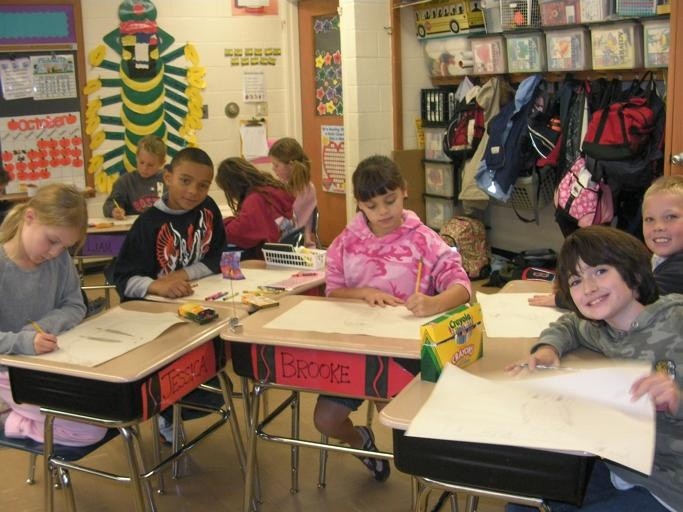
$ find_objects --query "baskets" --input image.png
[495,0,542,34]
[488,168,553,211]
[261,248,327,270]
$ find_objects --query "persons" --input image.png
[267,136,319,249]
[499,225,682,510]
[104,136,169,222]
[527,174,683,311]
[114,147,234,449]
[0,183,109,449]
[214,155,294,263]
[311,154,473,482]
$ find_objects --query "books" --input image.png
[424,91,460,122]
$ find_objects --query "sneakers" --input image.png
[354,425,390,482]
[157,416,174,446]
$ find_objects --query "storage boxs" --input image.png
[415,0,669,71]
[420,159,455,198]
[420,195,455,233]
[420,121,455,162]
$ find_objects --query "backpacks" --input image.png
[440,217,491,279]
[442,77,484,162]
[527,72,665,196]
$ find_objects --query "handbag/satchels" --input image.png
[553,157,613,237]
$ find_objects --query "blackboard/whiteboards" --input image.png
[0,43,96,203]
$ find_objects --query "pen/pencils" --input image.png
[515,361,577,372]
[112,197,128,221]
[223,292,239,302]
[242,290,260,296]
[205,291,221,301]
[292,272,318,277]
[415,256,424,293]
[212,292,228,300]
[190,283,198,287]
[27,317,60,350]
[257,285,285,291]
